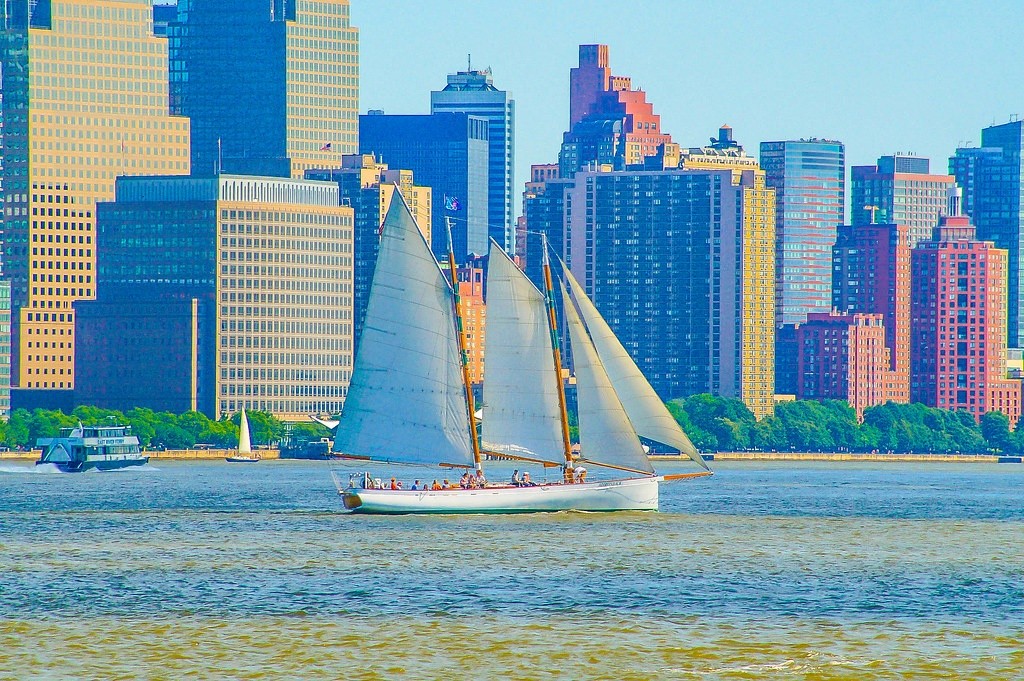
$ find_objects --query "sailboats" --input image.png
[323,179,716,515]
[224,404,259,463]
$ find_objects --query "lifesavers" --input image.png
[360,478,372,488]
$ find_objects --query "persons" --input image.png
[511,470,523,487]
[362,469,485,491]
[520,471,531,487]
[572,467,587,484]
[526,472,536,486]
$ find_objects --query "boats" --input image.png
[34,420,152,474]
